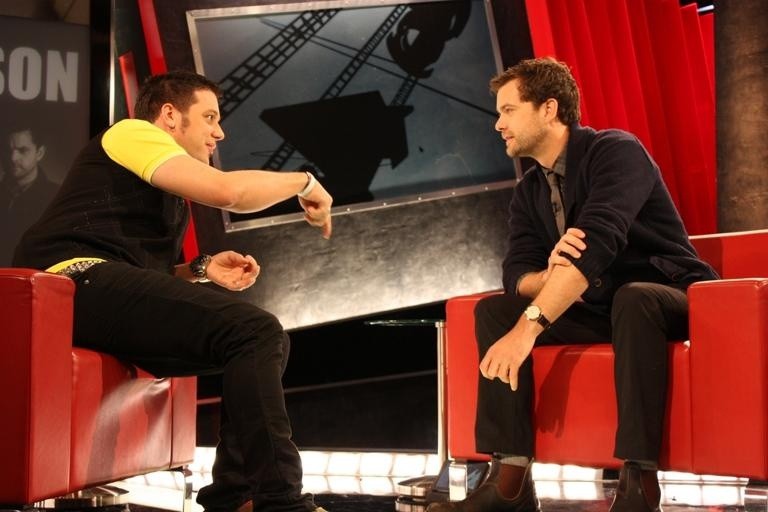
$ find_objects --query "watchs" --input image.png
[524,303,551,331]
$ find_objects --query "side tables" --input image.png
[362,317,449,500]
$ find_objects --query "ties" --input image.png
[546,172,565,237]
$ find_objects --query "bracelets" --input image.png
[189,253,211,283]
[297,171,315,197]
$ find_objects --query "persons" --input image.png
[426,58,721,512]
[13,70,333,512]
[0,123,63,269]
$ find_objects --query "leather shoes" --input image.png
[606,461,662,512]
[424,461,541,512]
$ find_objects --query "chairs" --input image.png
[0,264,199,511]
[444,230,768,512]
